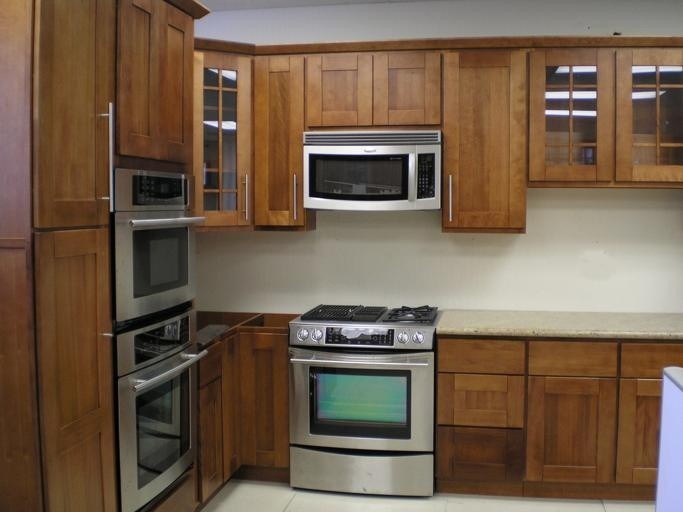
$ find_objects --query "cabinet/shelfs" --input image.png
[116,0,194,165]
[193,50,252,228]
[440,50,528,230]
[305,50,440,126]
[437,337,682,485]
[529,48,683,183]
[198,334,288,503]
[253,55,304,226]
[33,1,116,229]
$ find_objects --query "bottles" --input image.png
[581,148,592,164]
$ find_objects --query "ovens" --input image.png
[286,346,435,499]
[110,166,209,512]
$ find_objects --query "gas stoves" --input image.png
[288,303,439,349]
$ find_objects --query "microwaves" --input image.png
[301,130,443,212]
